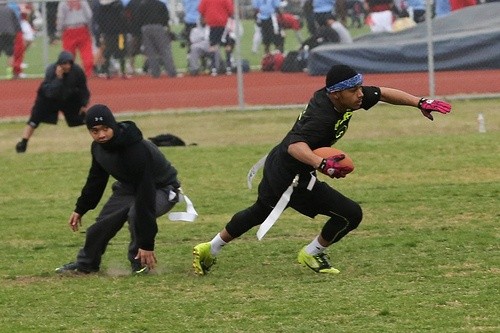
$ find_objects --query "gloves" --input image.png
[418,96,452,121]
[317,154,354,179]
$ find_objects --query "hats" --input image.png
[86,104,117,129]
[326,64,364,94]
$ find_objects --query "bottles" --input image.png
[476,113,487,132]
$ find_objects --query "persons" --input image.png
[16,51,91,153]
[250,0,481,59]
[192,65,452,276]
[46,0,177,79]
[56,104,180,275]
[182,0,234,76]
[0,0,41,79]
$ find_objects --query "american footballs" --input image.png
[313,147,355,174]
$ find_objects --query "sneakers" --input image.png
[54,261,100,274]
[297,246,341,275]
[131,261,151,274]
[193,242,217,276]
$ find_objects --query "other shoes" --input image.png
[16,138,28,152]
